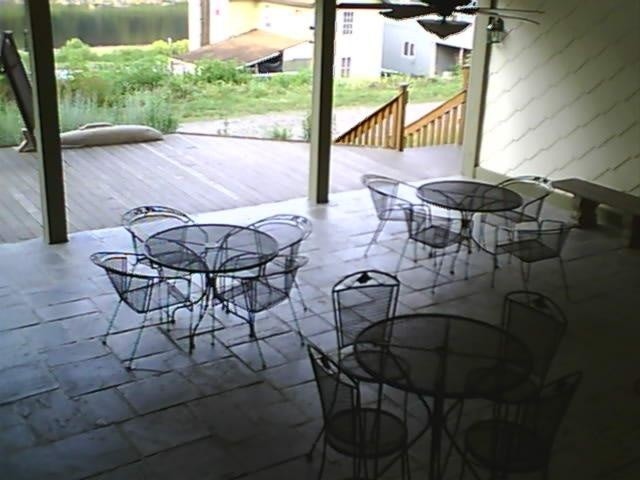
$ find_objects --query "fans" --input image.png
[335,0,544,27]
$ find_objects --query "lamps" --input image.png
[416,14,473,40]
[487,19,508,45]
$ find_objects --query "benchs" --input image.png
[552,177,640,247]
